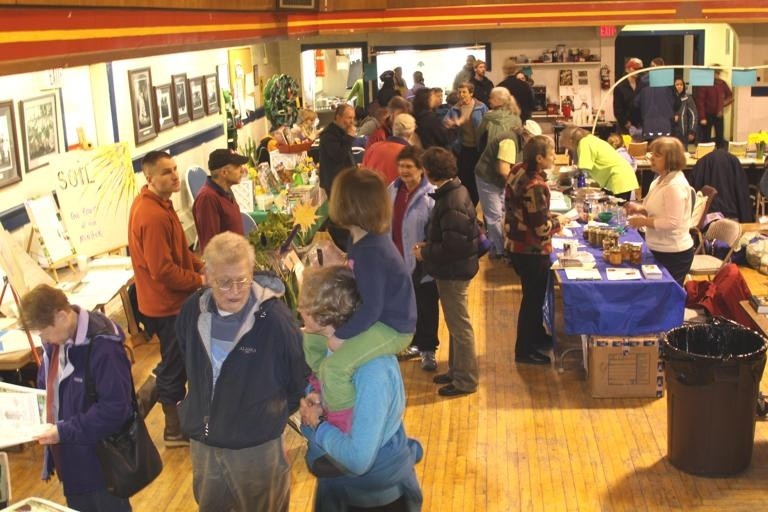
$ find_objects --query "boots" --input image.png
[134,372,191,447]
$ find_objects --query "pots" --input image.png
[568,184,610,224]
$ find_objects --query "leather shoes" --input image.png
[516,351,550,364]
[439,385,463,394]
[433,374,453,383]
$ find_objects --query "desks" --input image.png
[0,255,148,389]
[248,184,330,247]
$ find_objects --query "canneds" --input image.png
[588,226,641,266]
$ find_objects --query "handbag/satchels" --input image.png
[96,415,163,497]
[477,224,491,256]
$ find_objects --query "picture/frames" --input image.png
[0,92,60,191]
[128,66,220,147]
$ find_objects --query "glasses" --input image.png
[215,278,253,290]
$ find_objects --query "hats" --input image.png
[394,115,415,134]
[524,119,542,136]
[208,149,249,169]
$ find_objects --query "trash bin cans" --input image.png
[663,323,768,479]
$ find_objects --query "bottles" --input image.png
[586,227,642,267]
[264,156,320,191]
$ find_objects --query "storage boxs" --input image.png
[584,330,664,400]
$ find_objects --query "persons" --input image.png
[128,150,209,445]
[625,134,696,287]
[319,55,580,369]
[174,230,306,512]
[18,282,162,511]
[191,147,249,255]
[410,145,481,395]
[299,265,424,511]
[613,55,734,143]
[557,125,638,207]
[304,166,418,431]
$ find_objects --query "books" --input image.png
[750,295,768,314]
[0,390,42,453]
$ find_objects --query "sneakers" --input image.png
[397,345,420,360]
[421,351,436,370]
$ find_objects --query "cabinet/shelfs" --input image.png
[514,60,603,120]
